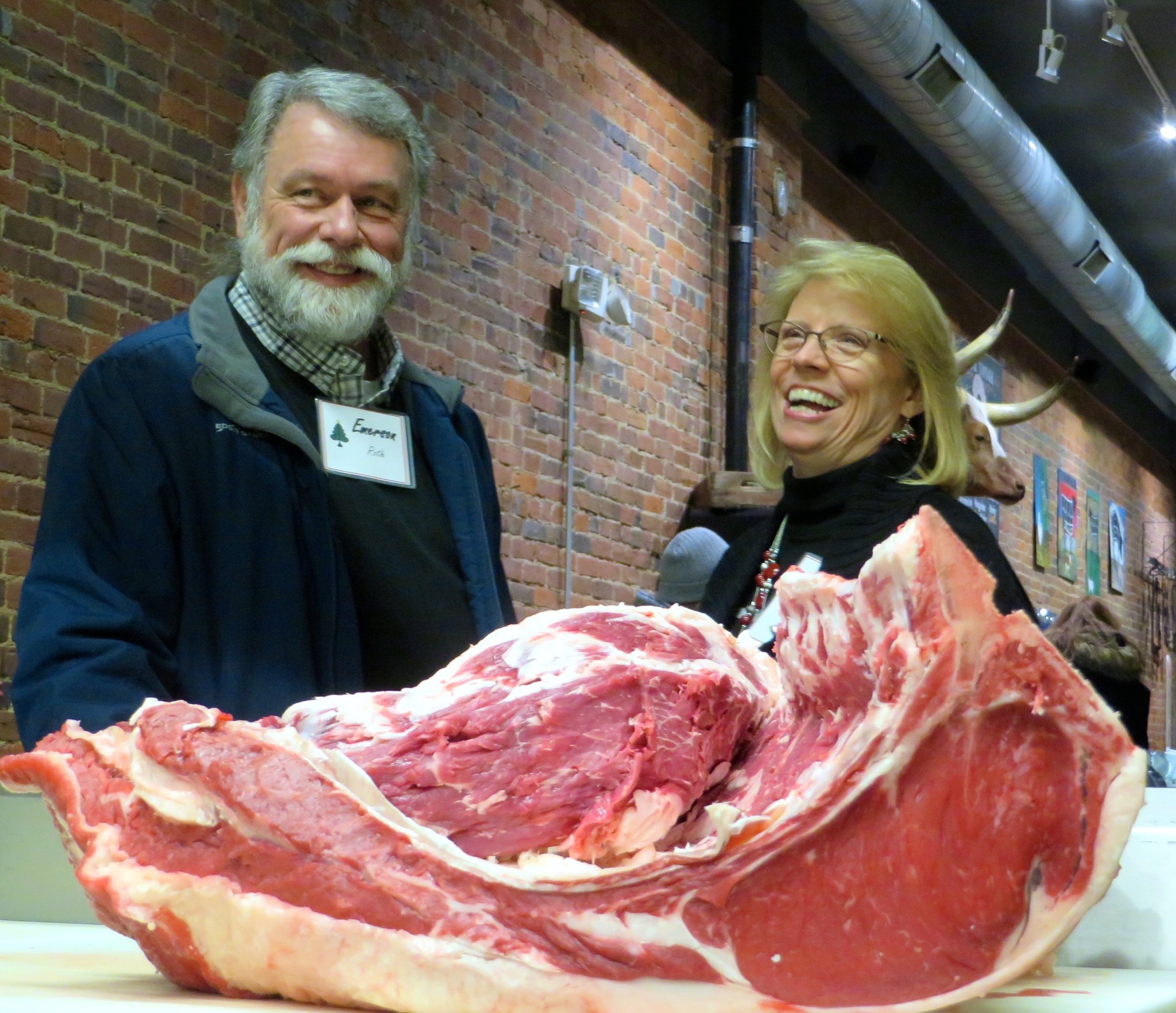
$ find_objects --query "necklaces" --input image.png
[736,510,791,640]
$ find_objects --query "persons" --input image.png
[636,527,729,614]
[1043,595,1150,753]
[11,65,515,753]
[706,236,1042,658]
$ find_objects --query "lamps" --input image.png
[561,262,633,326]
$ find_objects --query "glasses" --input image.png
[758,319,901,362]
